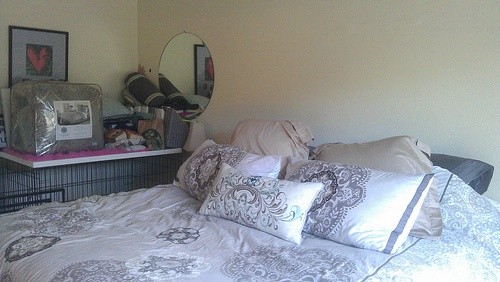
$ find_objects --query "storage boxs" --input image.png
[161,107,190,148]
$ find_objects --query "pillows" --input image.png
[195,160,327,245]
[126,72,166,108]
[172,139,283,203]
[230,119,315,178]
[158,73,188,111]
[310,136,443,240]
[282,156,436,254]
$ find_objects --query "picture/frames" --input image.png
[9,26,70,89]
[193,43,214,99]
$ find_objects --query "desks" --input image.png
[0,146,180,216]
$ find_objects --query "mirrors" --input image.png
[157,31,215,120]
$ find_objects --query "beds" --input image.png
[0,120,500,281]
